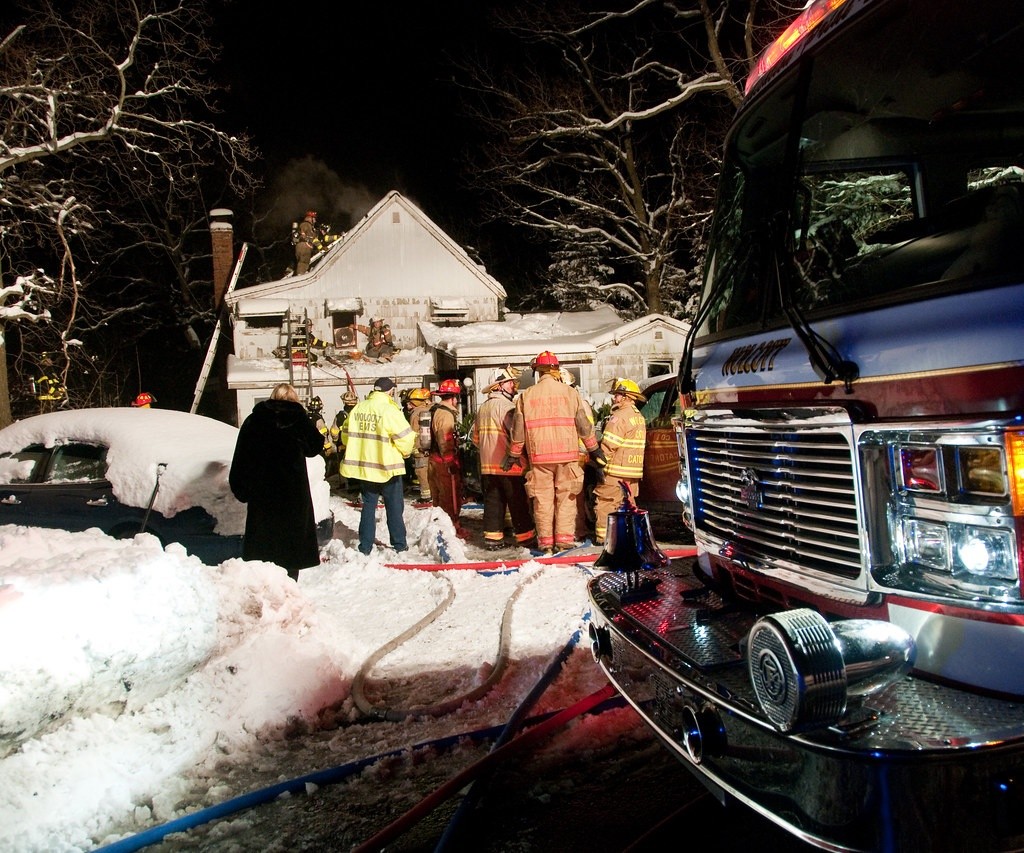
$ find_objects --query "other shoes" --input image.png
[483,537,506,552]
[414,498,434,510]
[513,535,538,549]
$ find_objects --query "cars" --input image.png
[582,371,688,534]
[1,442,335,568]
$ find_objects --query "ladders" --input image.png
[190,242,248,414]
[286,309,314,407]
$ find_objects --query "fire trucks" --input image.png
[579,0,1024,853]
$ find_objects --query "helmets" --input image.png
[340,391,358,406]
[610,378,642,393]
[421,388,432,397]
[487,363,517,385]
[309,396,323,410]
[407,388,429,399]
[439,379,461,393]
[529,351,559,369]
[35,351,52,362]
[135,393,152,406]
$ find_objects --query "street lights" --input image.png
[464,378,474,417]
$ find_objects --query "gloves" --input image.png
[448,463,459,475]
[323,442,333,457]
[589,447,607,468]
[500,449,522,472]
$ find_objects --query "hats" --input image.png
[373,377,397,392]
[398,390,407,397]
[608,378,647,403]
[558,367,575,386]
[481,369,523,394]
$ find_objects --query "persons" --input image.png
[473,351,599,555]
[309,397,332,480]
[331,377,468,556]
[132,393,151,408]
[35,351,68,410]
[229,383,325,581]
[596,379,645,546]
